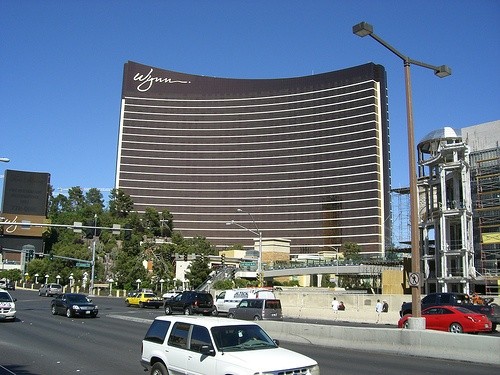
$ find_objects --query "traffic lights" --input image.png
[29,249,33,258]
[201,253,204,263]
[257,274,261,283]
[231,269,235,279]
[221,254,225,263]
[184,252,187,261]
[49,252,54,261]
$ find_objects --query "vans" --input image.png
[212,288,277,316]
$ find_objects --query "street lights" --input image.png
[351,22,452,318]
[226,209,262,287]
[319,245,339,288]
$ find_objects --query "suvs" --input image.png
[163,290,214,316]
[227,298,283,322]
[38,283,62,297]
[140,314,321,375]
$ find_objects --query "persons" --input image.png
[472,294,483,304]
[382,300,389,312]
[376,300,383,312]
[338,302,345,311]
[332,297,339,311]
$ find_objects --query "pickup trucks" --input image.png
[400,293,500,331]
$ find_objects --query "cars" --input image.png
[162,291,183,300]
[125,291,164,309]
[0,279,15,291]
[50,293,99,318]
[398,305,492,334]
[0,288,18,321]
